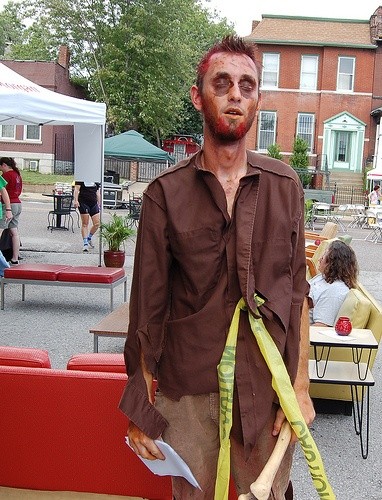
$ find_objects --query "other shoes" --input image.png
[82,243,88,251]
[7,259,19,265]
[88,240,95,247]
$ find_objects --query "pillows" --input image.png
[66,352,127,373]
[333,289,372,329]
[0,346,51,369]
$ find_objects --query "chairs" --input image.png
[307,199,382,243]
[126,192,143,220]
[46,189,80,234]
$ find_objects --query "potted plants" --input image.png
[97,212,137,268]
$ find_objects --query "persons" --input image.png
[367,185,380,227]
[73,181,101,251]
[0,175,13,283]
[308,239,359,328]
[0,157,22,265]
[119,33,316,500]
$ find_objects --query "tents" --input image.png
[0,61,107,268]
[367,166,382,205]
[104,130,175,169]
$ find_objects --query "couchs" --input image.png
[307,281,382,416]
[304,222,353,308]
[0,366,239,500]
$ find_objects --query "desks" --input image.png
[41,193,80,230]
[119,201,142,216]
[90,303,130,353]
[309,359,375,459]
[309,326,379,382]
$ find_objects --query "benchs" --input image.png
[0,263,128,313]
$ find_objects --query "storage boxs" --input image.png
[103,187,123,208]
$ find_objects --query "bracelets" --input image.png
[6,208,13,211]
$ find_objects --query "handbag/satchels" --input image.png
[0,228,13,262]
